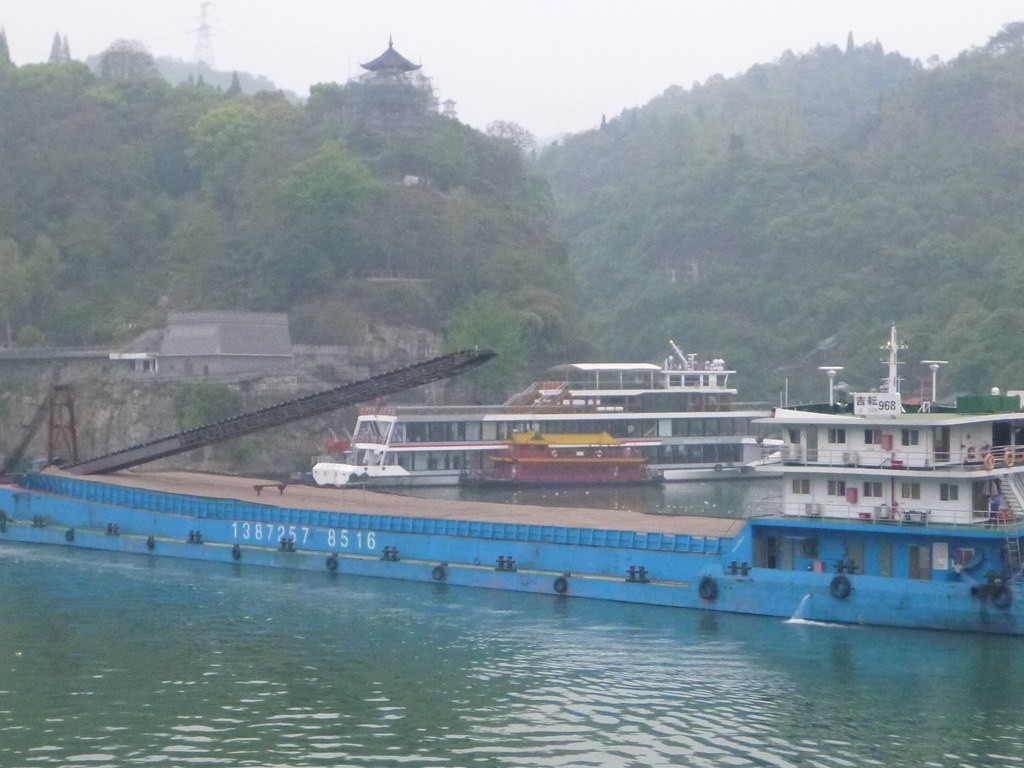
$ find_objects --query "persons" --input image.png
[627,422,634,438]
[985,492,1001,529]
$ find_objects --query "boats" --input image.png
[455,430,667,491]
[312,338,804,490]
[24,344,1023,636]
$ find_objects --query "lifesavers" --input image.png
[554,578,567,592]
[993,585,1013,608]
[699,576,716,599]
[232,547,241,559]
[727,461,733,467]
[65,530,73,541]
[984,453,995,470]
[831,576,850,597]
[715,464,722,472]
[326,557,337,570]
[147,539,154,550]
[1003,451,1015,466]
[432,566,443,580]
